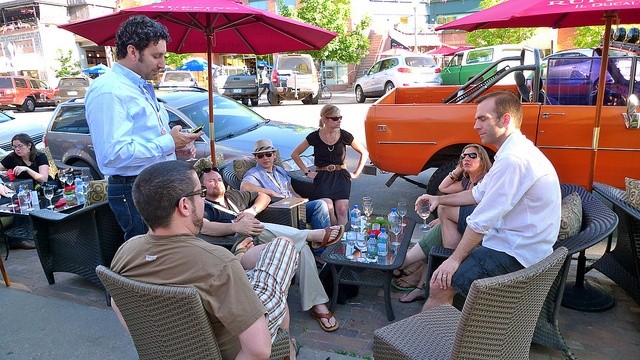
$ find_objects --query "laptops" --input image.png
[268,197,309,209]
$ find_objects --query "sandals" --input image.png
[400,286,427,303]
[381,267,405,279]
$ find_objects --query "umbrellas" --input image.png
[434,0,640,290]
[176,57,216,85]
[81,60,111,76]
[56,0,338,170]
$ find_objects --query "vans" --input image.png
[440,45,542,84]
[267,55,320,105]
[0,76,54,112]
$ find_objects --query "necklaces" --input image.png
[323,128,339,153]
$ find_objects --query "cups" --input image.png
[31,191,38,205]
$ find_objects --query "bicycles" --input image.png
[321,79,332,99]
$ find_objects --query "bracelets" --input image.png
[448,171,459,182]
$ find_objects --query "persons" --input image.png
[240,139,332,228]
[109,159,299,360]
[0,133,50,184]
[414,91,563,312]
[84,17,206,241]
[391,143,492,304]
[195,168,345,333]
[258,63,274,101]
[291,105,368,227]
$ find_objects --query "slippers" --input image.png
[311,225,344,249]
[391,277,417,291]
[309,308,339,332]
[288,337,299,360]
[230,234,253,256]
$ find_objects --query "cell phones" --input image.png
[191,125,204,132]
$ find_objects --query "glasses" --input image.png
[325,116,343,120]
[256,152,275,158]
[460,153,481,159]
[176,185,207,207]
[12,144,26,148]
[198,167,220,180]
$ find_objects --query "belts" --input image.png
[315,164,347,172]
[108,174,137,183]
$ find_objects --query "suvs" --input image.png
[212,67,259,106]
[354,54,441,103]
[54,77,90,106]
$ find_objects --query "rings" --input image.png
[442,275,447,278]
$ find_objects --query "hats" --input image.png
[252,140,277,155]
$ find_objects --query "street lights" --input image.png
[401,7,417,53]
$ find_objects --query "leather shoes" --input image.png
[9,241,36,250]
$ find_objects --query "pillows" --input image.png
[233,148,284,181]
[84,179,108,208]
[557,191,584,242]
[624,177,640,211]
[193,151,225,175]
[39,146,58,180]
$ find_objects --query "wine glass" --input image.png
[44,187,56,209]
[59,169,67,190]
[346,232,357,260]
[363,202,374,225]
[362,196,372,217]
[280,180,290,205]
[397,202,408,228]
[416,201,432,232]
[65,173,75,188]
[392,222,401,246]
[357,234,368,261]
[24,190,33,211]
[4,182,16,209]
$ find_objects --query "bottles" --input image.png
[350,204,361,229]
[75,175,85,205]
[376,227,389,256]
[388,208,400,234]
[18,189,28,212]
[17,185,24,193]
[366,233,378,262]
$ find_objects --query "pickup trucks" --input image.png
[365,28,640,219]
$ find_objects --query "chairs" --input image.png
[27,201,125,307]
[95,266,289,360]
[585,180,640,306]
[221,155,318,229]
[423,184,619,360]
[0,147,90,233]
[373,246,569,360]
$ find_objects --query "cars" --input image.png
[43,86,316,182]
[541,49,640,82]
[159,71,198,90]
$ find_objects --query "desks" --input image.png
[1,193,87,261]
[321,213,416,321]
